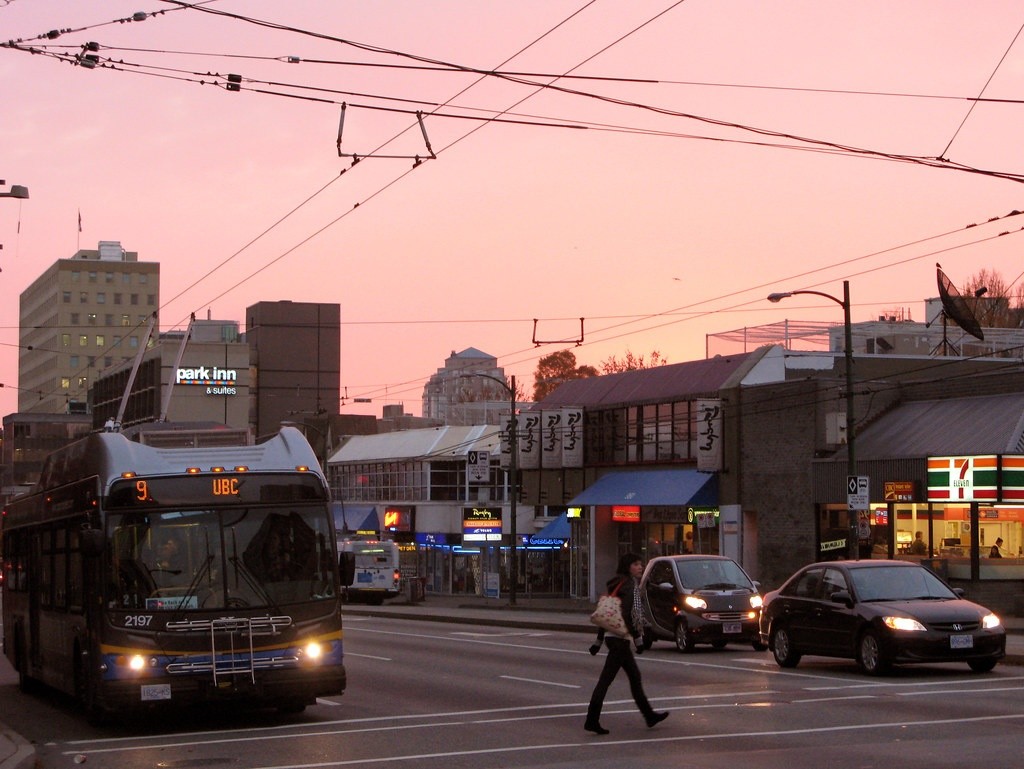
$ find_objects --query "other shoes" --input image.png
[644,711,670,729]
[583,718,610,735]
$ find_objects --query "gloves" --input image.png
[635,644,644,655]
[588,643,600,657]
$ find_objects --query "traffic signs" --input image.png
[846,474,869,514]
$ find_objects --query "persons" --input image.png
[245,530,302,584]
[911,531,927,554]
[140,536,194,587]
[991,536,1004,558]
[584,553,669,734]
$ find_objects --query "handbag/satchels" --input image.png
[591,579,629,637]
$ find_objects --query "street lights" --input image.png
[279,420,329,481]
[461,373,517,605]
[768,280,860,559]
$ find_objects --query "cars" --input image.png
[759,559,1007,679]
[638,554,767,654]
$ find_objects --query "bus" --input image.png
[0,309,351,731]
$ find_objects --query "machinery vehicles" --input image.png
[315,499,401,605]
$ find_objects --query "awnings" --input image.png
[532,512,570,538]
[334,506,379,530]
[566,469,719,506]
